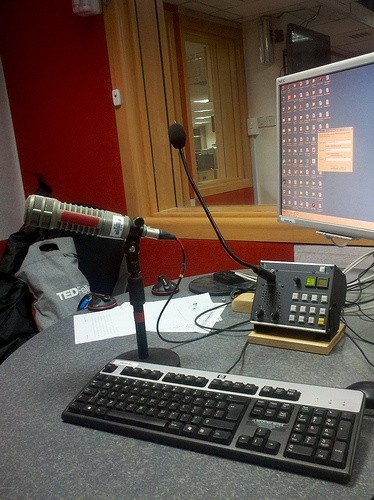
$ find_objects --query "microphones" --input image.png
[168,123,276,282]
[23,194,175,242]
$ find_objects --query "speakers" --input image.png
[286,23,331,75]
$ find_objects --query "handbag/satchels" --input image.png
[15,237,91,332]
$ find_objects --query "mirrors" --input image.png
[135,0,374,212]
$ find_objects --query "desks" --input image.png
[1,267,374,500]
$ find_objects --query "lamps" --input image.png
[72,0,102,17]
[257,16,274,66]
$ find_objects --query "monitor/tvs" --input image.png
[275,52,373,240]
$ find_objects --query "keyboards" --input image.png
[61,358,368,485]
